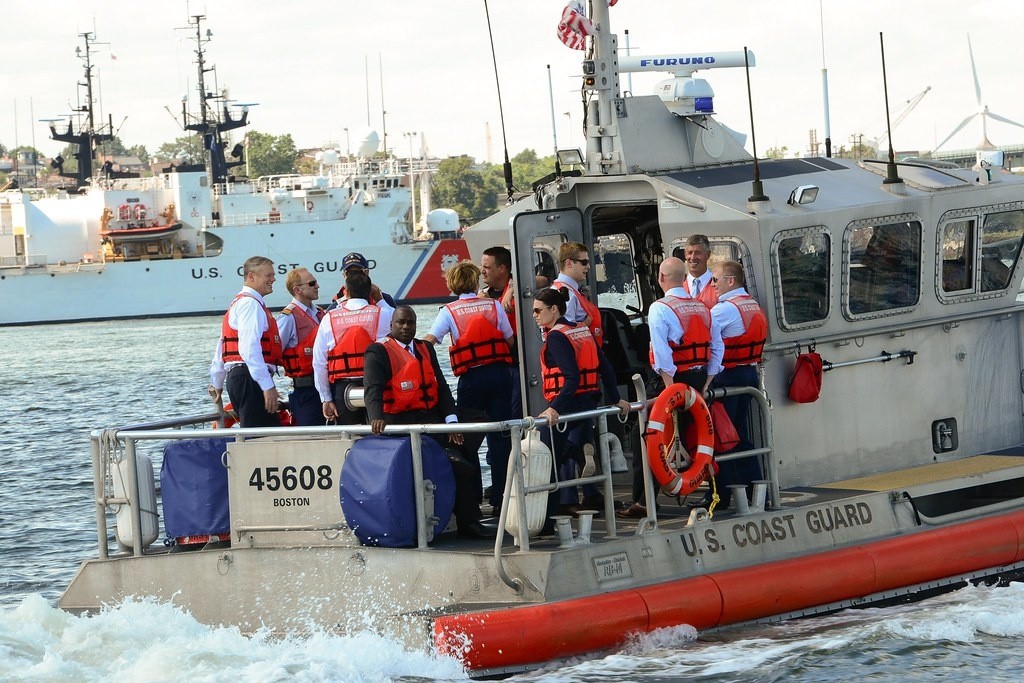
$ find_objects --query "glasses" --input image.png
[563,258,589,266]
[532,306,550,313]
[713,276,736,282]
[297,279,318,286]
[345,269,367,276]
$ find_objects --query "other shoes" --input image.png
[484,486,494,499]
[580,443,596,477]
[456,521,497,540]
[493,506,501,516]
[615,502,647,518]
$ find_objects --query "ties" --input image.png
[405,345,409,351]
[578,285,587,297]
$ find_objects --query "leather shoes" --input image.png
[687,497,733,510]
[747,501,769,511]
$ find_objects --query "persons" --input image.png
[682,234,718,309]
[275,267,326,426]
[476,246,548,336]
[550,242,623,518]
[687,260,768,511]
[533,286,631,535]
[312,252,397,426]
[422,262,514,517]
[615,257,712,518]
[209,257,285,429]
[363,306,497,540]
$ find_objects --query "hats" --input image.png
[342,252,368,270]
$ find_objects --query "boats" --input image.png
[52,0,1022,681]
[0,0,445,329]
[100,221,184,246]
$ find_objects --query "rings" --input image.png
[458,434,461,436]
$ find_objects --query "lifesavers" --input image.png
[307,201,313,210]
[645,382,716,497]
[120,204,131,219]
[134,203,146,219]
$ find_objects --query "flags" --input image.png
[557,0,598,51]
[111,54,116,60]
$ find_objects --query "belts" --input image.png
[337,378,362,382]
[227,363,274,377]
